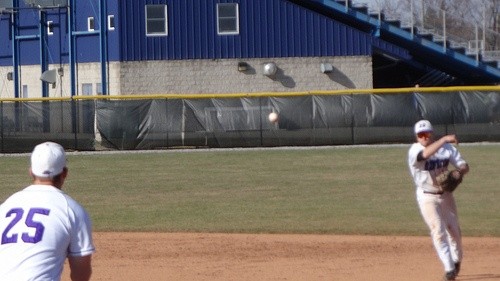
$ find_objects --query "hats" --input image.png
[30,141,65,178]
[414,119,432,134]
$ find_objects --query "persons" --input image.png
[408,120,470,281]
[0,142,96,281]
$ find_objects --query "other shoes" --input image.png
[454,262,460,276]
[447,271,456,281]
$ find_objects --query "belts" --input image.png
[423,191,445,195]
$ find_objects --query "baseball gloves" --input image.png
[436,169,462,192]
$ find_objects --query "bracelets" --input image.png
[457,169,464,177]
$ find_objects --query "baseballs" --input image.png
[268,112,279,123]
